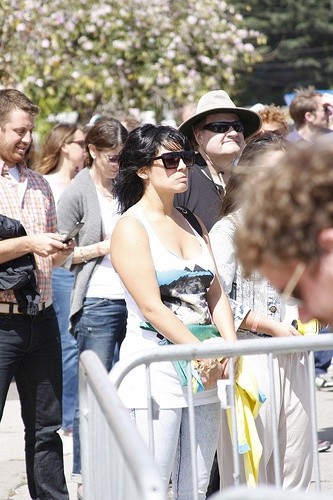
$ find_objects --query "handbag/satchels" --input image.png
[139,322,225,393]
[0,214,38,304]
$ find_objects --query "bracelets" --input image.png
[78,245,87,264]
[250,313,262,333]
[95,241,103,256]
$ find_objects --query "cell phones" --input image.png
[57,222,84,243]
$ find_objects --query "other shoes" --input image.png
[77,484,83,500]
[318,439,331,452]
[60,435,73,455]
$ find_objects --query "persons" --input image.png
[0,84,333,500]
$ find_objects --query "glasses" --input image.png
[200,120,245,133]
[279,261,305,309]
[101,150,119,162]
[68,140,85,148]
[146,150,196,169]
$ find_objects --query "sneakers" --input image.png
[315,374,333,392]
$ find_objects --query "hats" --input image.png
[176,90,262,152]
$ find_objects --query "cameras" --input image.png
[291,318,319,335]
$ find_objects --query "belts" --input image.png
[0,297,52,316]
